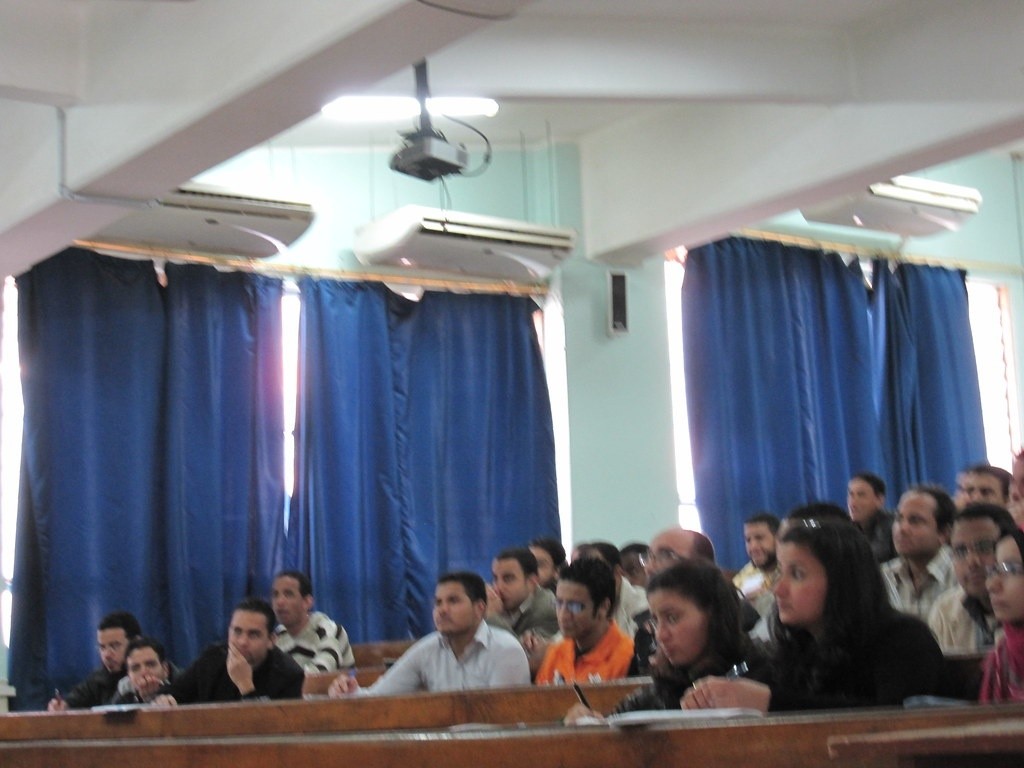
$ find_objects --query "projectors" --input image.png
[389,136,468,181]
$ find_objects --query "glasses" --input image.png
[638,548,685,568]
[952,540,996,559]
[984,562,1023,576]
[550,599,586,613]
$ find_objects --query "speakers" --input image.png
[612,273,627,332]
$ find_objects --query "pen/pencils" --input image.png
[54,688,63,705]
[346,665,357,684]
[571,680,594,710]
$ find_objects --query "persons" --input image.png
[268,568,357,677]
[327,451,1024,727]
[45,611,148,711]
[183,599,305,704]
[111,639,186,709]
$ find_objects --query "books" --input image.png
[607,707,766,729]
